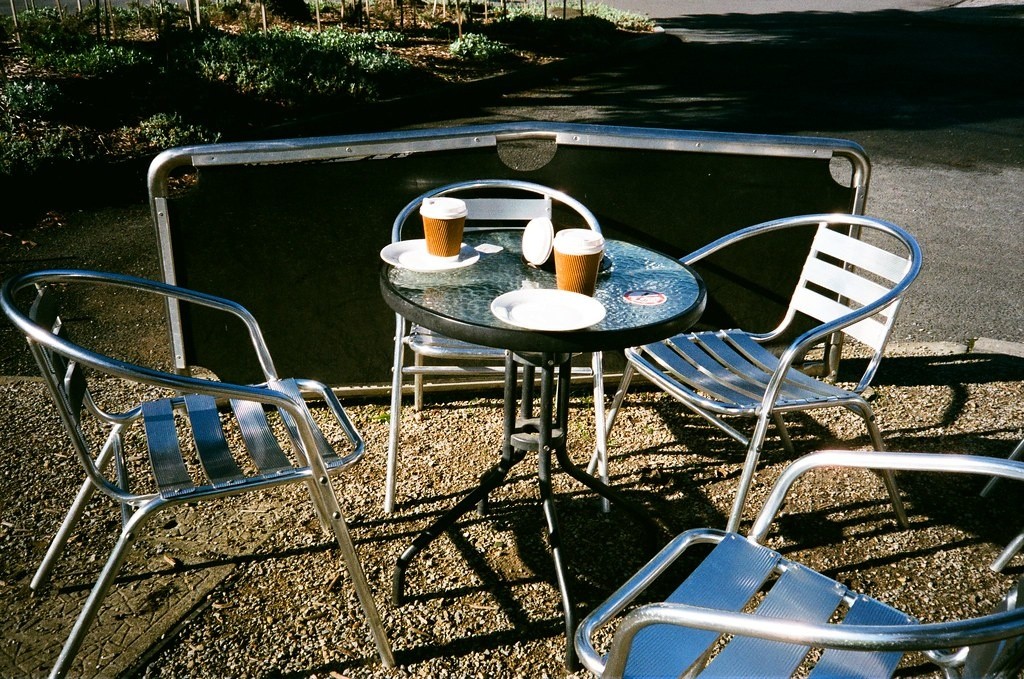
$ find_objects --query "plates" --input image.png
[380,237,480,272]
[490,287,607,332]
[520,244,612,276]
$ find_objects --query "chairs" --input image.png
[587,213,923,539]
[574,451,1024,679]
[384,179,611,514]
[0,270,394,679]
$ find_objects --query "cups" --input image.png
[419,197,467,262]
[554,228,605,300]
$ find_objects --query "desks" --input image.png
[379,238,707,672]
